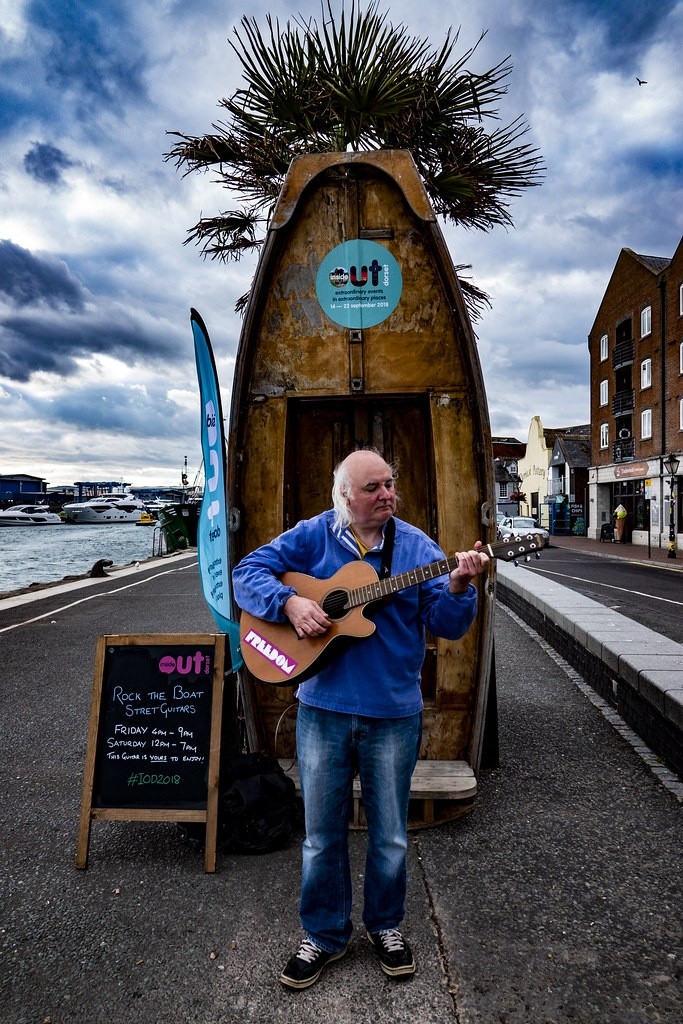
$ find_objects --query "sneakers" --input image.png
[366,920,416,977]
[281,921,354,990]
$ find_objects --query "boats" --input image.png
[61,492,147,524]
[135,499,181,526]
[0,504,62,527]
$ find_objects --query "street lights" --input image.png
[663,451,680,558]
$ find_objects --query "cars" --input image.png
[497,515,550,549]
[496,511,506,537]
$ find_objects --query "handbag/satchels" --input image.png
[179,757,296,853]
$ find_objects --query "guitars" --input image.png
[238,532,546,687]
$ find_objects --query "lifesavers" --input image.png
[619,428,630,439]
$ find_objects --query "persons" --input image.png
[232,448,490,988]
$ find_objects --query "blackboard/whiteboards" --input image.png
[80,633,228,822]
